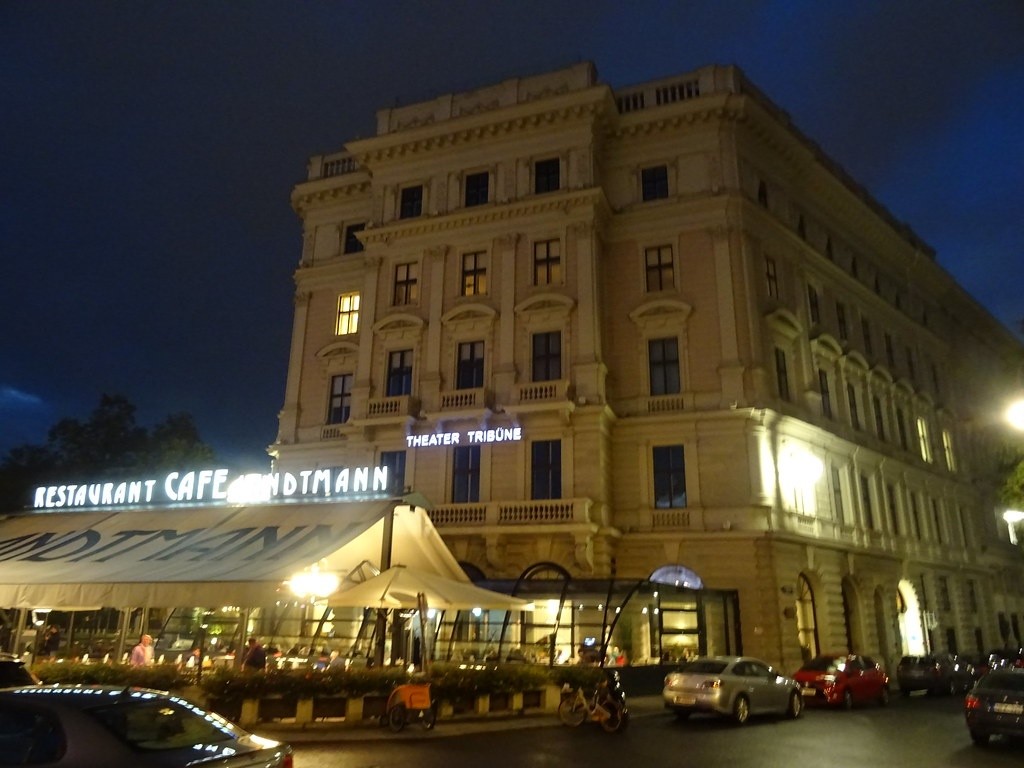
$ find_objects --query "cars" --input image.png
[663,656,806,724]
[791,652,890,711]
[897,648,1024,747]
[0,654,296,768]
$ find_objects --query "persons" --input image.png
[184,646,204,667]
[45,625,61,658]
[450,628,718,700]
[130,634,154,669]
[207,634,400,676]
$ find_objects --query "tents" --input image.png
[1,499,478,606]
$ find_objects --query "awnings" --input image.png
[312,564,530,613]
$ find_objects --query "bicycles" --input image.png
[387,677,440,731]
[556,670,628,732]
